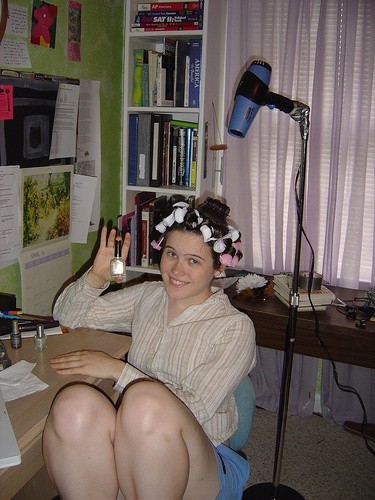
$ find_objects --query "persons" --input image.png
[41,196,258,500]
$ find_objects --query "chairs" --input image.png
[224,374,257,464]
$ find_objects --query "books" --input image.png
[128,214,137,266]
[137,196,161,266]
[151,195,167,264]
[141,206,150,266]
[127,112,198,192]
[117,210,136,229]
[130,1,203,32]
[131,37,202,109]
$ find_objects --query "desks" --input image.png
[0,318,133,499]
[228,270,375,369]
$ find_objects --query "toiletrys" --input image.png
[10,320,22,349]
[32,324,48,352]
[110,240,127,278]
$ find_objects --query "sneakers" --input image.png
[343,421,375,442]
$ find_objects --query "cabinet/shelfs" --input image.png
[120,0,223,275]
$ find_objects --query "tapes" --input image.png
[299,270,323,290]
